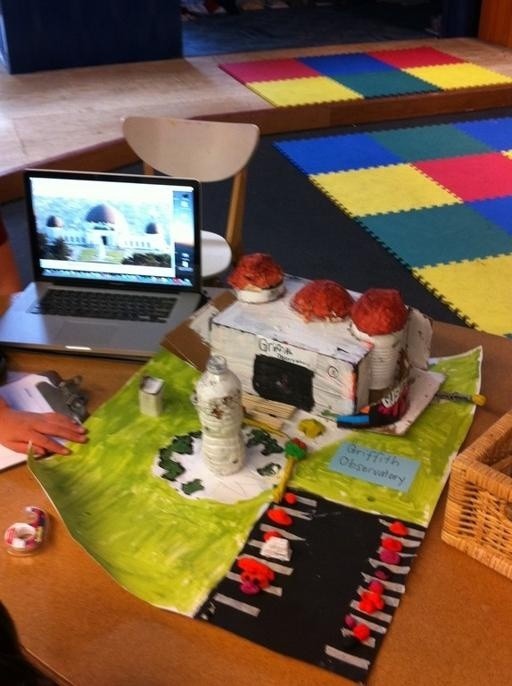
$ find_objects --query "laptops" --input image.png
[0,167,203,361]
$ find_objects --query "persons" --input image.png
[1,225,90,458]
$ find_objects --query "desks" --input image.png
[1,275,512,686]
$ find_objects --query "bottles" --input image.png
[194,355,248,476]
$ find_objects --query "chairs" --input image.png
[120,114,261,287]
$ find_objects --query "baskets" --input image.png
[441,409,511,580]
